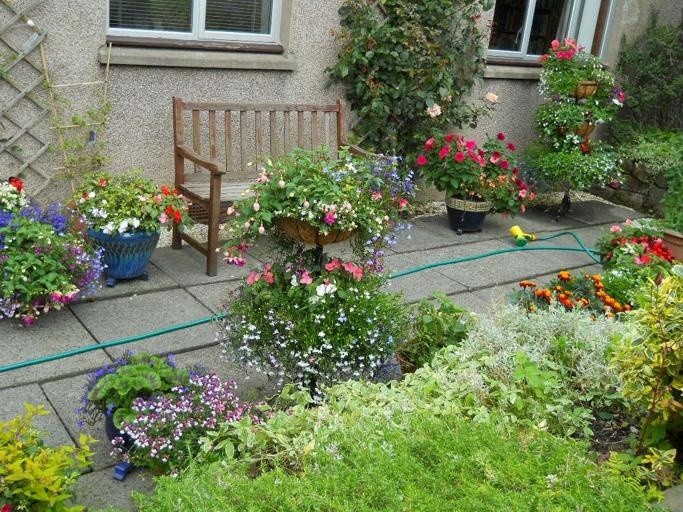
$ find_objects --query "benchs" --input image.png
[167,97,389,274]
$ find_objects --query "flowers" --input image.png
[75,352,271,479]
[521,38,627,222]
[518,220,675,319]
[216,148,418,400]
[0,171,190,330]
[413,131,528,214]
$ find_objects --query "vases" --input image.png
[444,194,492,235]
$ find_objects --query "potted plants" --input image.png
[396,288,479,376]
